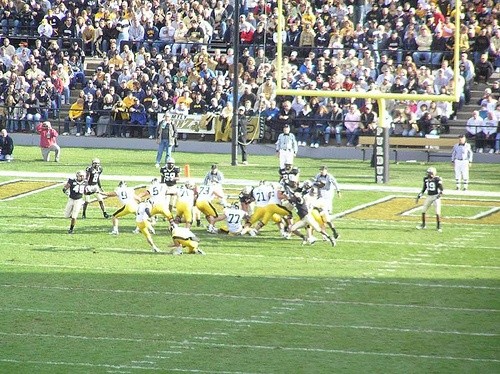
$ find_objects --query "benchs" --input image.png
[354,135,463,165]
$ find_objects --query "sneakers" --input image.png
[155,163,159,168]
[416,224,443,231]
[5,158,11,163]
[0,126,500,154]
[61,166,333,255]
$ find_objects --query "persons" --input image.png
[62,169,97,233]
[230,106,249,165]
[275,123,299,172]
[90,157,341,255]
[34,120,61,163]
[0,129,14,163]
[414,165,445,232]
[154,113,176,168]
[0,0,500,155]
[80,157,110,220]
[449,134,475,190]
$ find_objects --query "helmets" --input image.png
[426,167,437,174]
[166,157,175,164]
[284,159,293,165]
[92,158,100,164]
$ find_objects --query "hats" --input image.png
[283,123,290,128]
[164,112,173,117]
[45,121,51,126]
